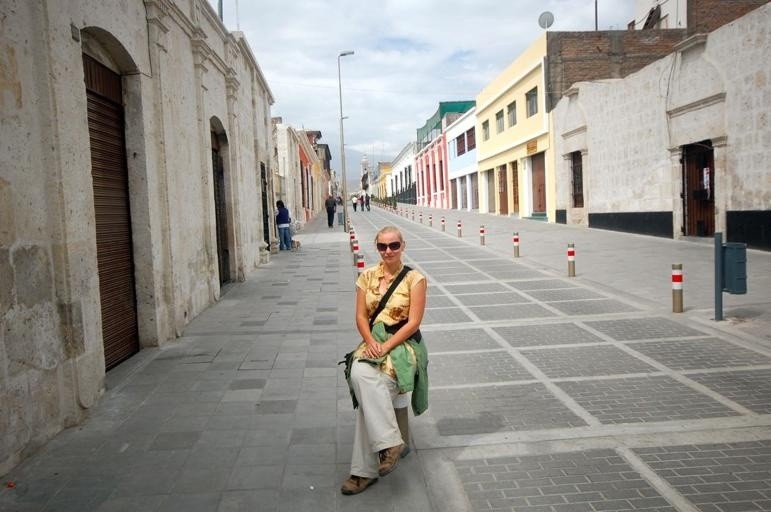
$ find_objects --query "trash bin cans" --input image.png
[714,239,746,295]
[337,213,344,225]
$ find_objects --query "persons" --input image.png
[351,195,357,211]
[325,195,337,228]
[364,193,371,211]
[331,195,343,213]
[335,202,344,225]
[357,195,365,211]
[273,199,292,251]
[338,224,430,498]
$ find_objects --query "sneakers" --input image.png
[377,443,411,477]
[341,476,377,496]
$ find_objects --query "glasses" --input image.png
[377,241,401,251]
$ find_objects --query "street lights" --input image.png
[337,48,354,230]
